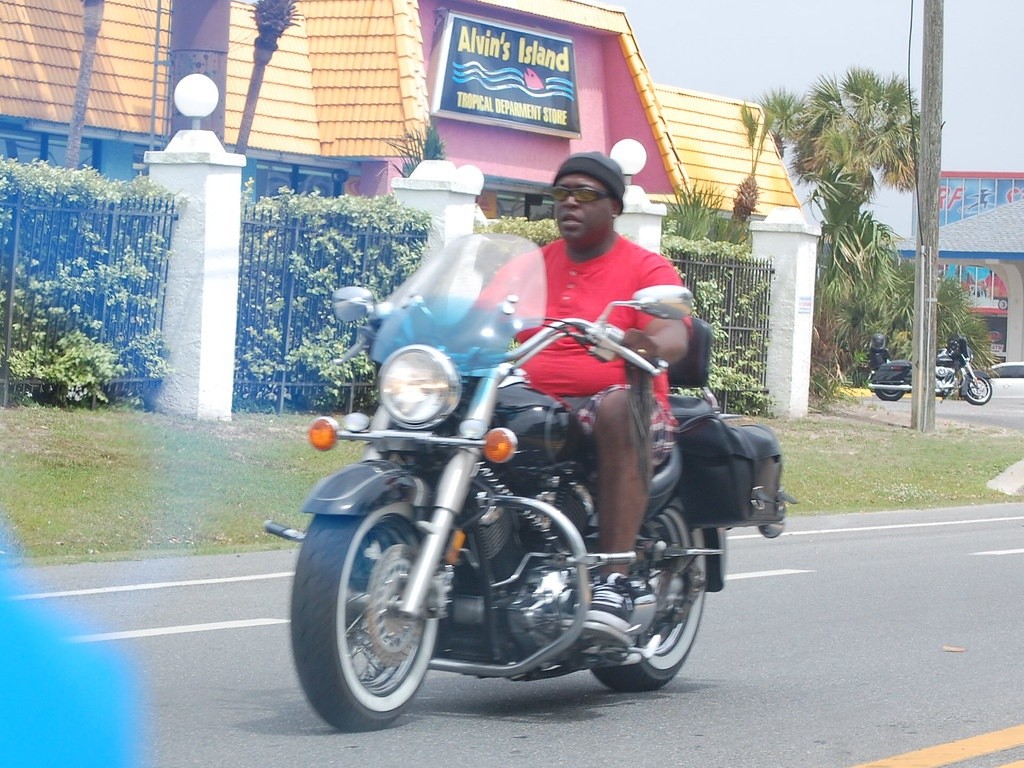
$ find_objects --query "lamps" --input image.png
[173,75,219,130]
[609,138,648,186]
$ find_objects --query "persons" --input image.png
[458,152,689,645]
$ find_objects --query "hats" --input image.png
[553,151,625,216]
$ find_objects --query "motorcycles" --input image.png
[263,231,794,732]
[869,338,994,404]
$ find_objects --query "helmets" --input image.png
[870,334,885,350]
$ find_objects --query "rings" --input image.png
[638,349,647,353]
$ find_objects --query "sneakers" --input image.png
[582,572,635,643]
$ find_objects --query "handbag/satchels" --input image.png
[678,415,798,531]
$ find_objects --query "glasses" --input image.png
[550,186,614,204]
